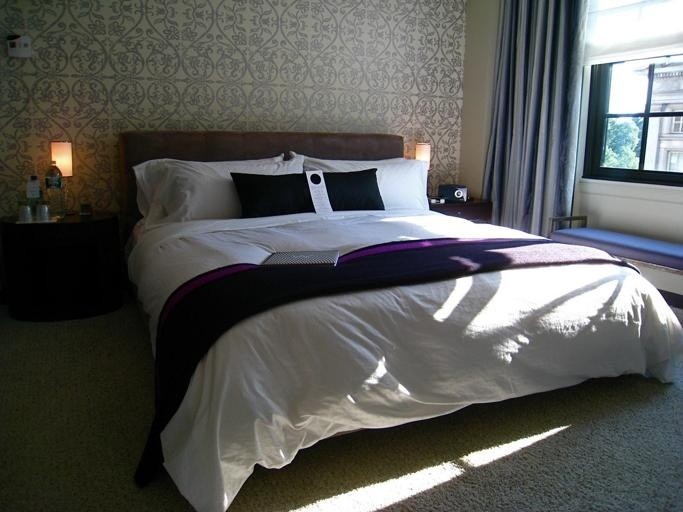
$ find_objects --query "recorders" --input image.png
[438,184,468,203]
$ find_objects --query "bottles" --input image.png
[25,175,43,218]
[45,160,65,220]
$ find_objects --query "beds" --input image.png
[123,129,667,510]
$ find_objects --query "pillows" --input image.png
[134,151,429,224]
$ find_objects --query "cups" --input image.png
[19,206,33,223]
[36,204,49,222]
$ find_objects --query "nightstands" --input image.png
[1,212,123,321]
[426,197,493,225]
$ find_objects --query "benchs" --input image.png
[546,211,682,299]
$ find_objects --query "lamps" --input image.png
[415,142,430,173]
[49,138,74,210]
